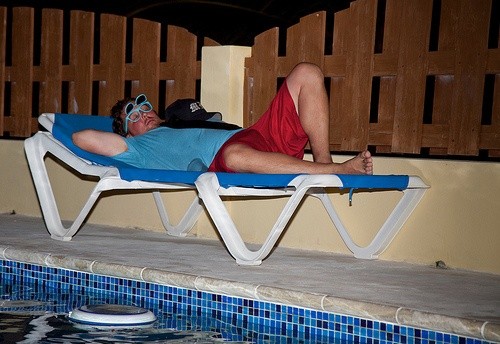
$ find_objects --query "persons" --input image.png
[71,62,374,175]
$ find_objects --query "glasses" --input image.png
[125,93,154,133]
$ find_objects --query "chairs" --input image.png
[23,112,431,266]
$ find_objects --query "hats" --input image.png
[164,99,222,121]
